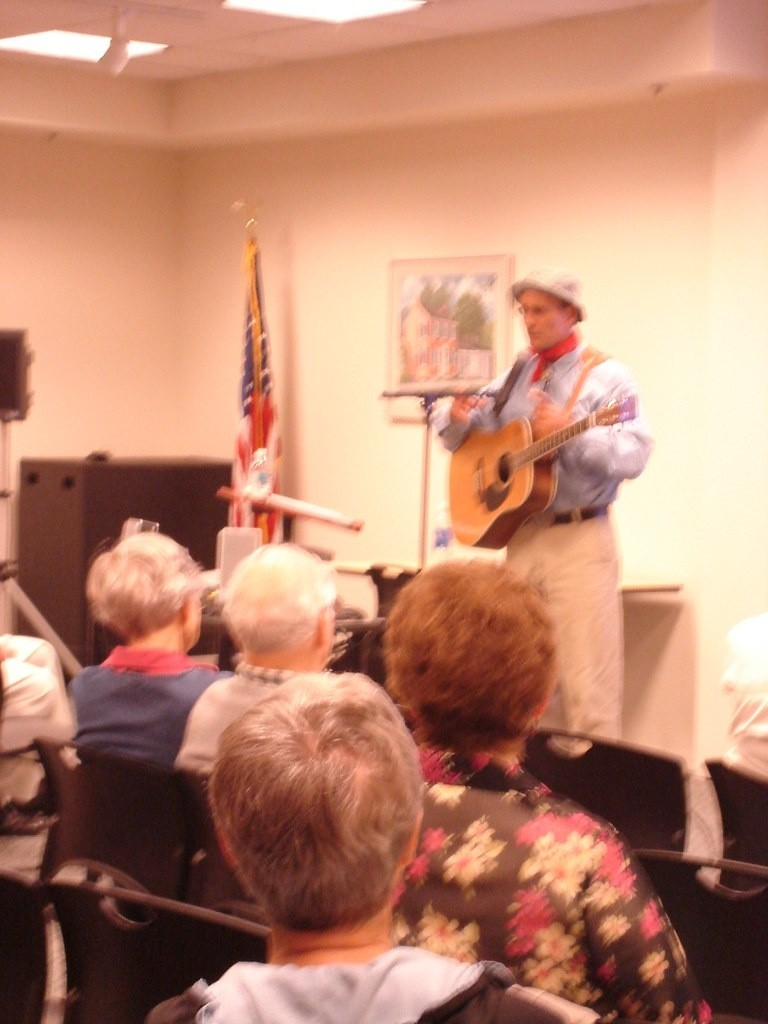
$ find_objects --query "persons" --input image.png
[386,556,714,1024]
[434,263,656,743]
[65,531,233,765]
[721,611,768,779]
[142,670,570,1024]
[0,633,115,1024]
[172,541,339,773]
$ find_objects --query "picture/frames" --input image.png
[388,252,518,422]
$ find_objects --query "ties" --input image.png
[529,332,578,383]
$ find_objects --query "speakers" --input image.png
[0,327,27,423]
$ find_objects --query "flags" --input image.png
[228,240,283,546]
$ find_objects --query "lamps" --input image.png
[95,5,133,82]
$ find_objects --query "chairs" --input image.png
[0,725,768,1024]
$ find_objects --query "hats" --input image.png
[510,268,584,322]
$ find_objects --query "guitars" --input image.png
[445,390,641,552]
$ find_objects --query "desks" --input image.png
[621,591,694,772]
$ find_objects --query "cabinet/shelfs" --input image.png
[19,456,234,689]
